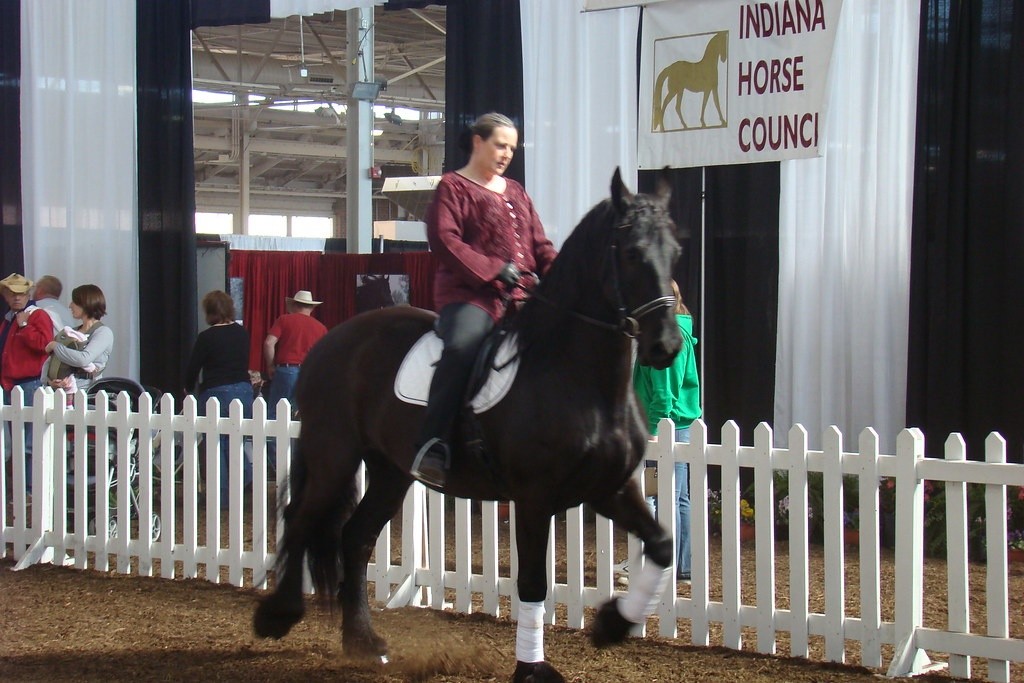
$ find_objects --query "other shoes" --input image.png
[244,481,252,513]
[415,451,448,489]
[611,559,629,578]
[199,499,206,509]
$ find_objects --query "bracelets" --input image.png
[18,322,28,328]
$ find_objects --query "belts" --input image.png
[275,364,302,367]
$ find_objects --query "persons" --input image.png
[613,283,701,583]
[1,273,328,508]
[414,112,560,490]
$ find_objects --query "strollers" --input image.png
[153,407,204,501]
[67,376,162,542]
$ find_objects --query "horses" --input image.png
[233,165,682,683]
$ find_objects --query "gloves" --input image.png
[494,264,520,291]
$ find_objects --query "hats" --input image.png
[0,273,33,293]
[286,290,324,307]
